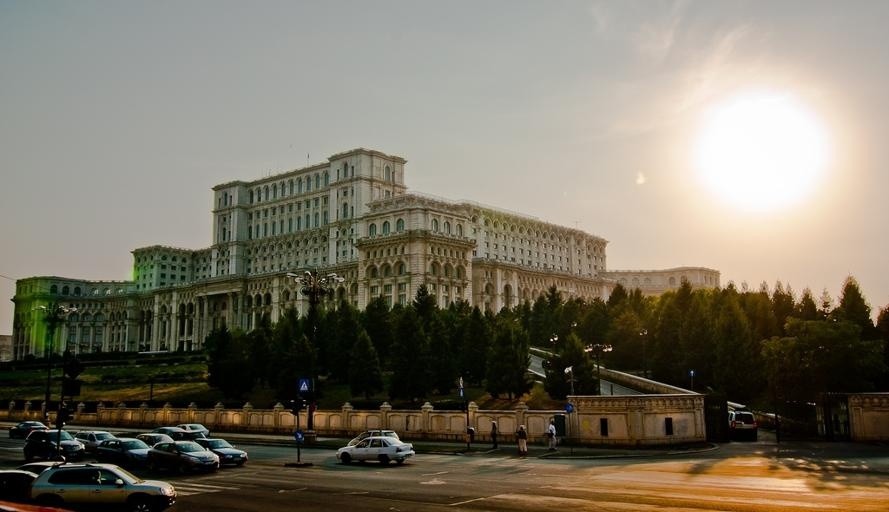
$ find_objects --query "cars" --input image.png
[347,427,399,447]
[335,436,416,465]
[0,418,251,511]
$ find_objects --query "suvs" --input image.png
[728,409,759,442]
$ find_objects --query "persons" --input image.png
[490,420,497,449]
[547,420,558,451]
[517,424,528,455]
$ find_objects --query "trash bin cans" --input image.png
[468,427,474,442]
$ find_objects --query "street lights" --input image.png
[570,320,577,333]
[639,326,650,378]
[285,269,346,445]
[31,304,80,424]
[549,331,560,356]
[582,342,614,396]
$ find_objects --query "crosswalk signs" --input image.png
[299,378,310,392]
